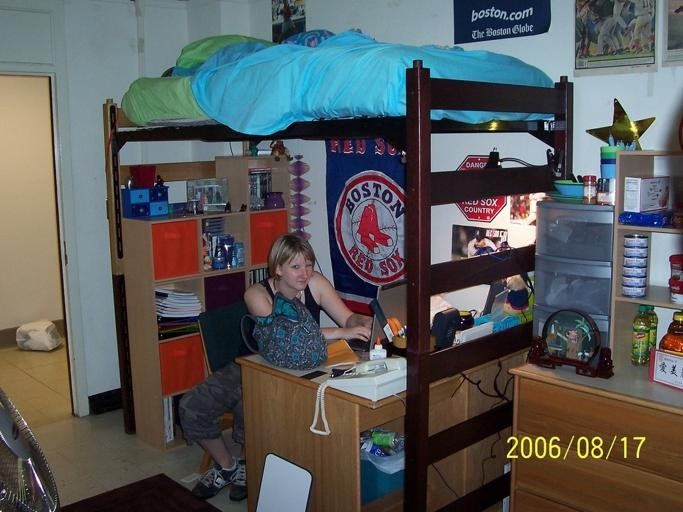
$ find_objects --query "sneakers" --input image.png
[193,461,238,500]
[230,456,247,501]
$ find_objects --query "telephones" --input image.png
[326,358,407,402]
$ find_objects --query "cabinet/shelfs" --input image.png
[531,200,614,362]
[121,156,292,452]
[509,356,683,511]
[608,151,683,369]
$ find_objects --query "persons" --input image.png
[179,234,373,501]
[276,0,299,44]
[575,0,654,58]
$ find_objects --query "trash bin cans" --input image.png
[360,446,405,506]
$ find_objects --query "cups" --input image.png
[600,145,625,178]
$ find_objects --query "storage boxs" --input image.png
[362,451,407,503]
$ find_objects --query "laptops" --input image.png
[346,278,407,352]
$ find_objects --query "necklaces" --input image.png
[273,279,302,301]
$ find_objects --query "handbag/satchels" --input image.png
[240,290,328,369]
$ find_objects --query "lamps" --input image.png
[483,146,565,178]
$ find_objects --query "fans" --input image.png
[0,386,60,512]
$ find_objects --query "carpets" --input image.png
[63,473,224,512]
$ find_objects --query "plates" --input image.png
[547,192,587,203]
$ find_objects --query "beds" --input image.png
[103,60,573,512]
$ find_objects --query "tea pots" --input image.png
[264,191,286,209]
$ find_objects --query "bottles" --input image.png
[632,305,683,367]
[584,175,614,206]
[212,235,244,268]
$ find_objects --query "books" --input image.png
[249,268,270,286]
[154,284,202,339]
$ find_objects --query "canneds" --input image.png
[584,176,597,205]
[621,232,649,297]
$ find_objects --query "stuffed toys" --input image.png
[503,274,530,316]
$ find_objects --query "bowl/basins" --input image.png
[554,179,588,194]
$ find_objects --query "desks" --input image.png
[234,319,531,512]
